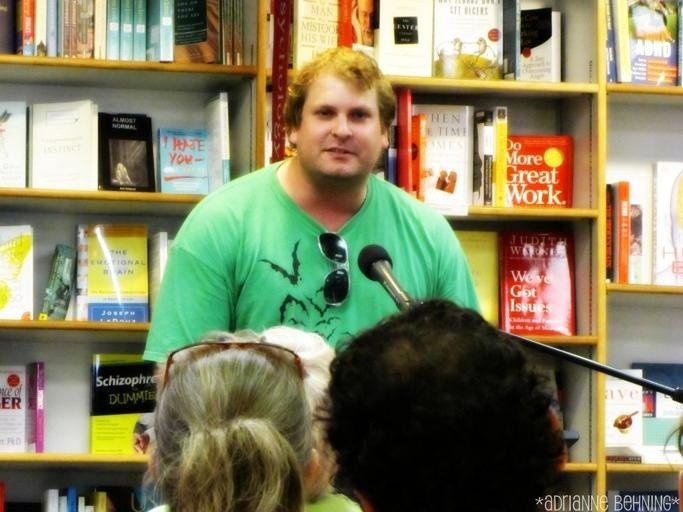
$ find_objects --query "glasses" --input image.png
[158,338,306,392]
[317,230,349,307]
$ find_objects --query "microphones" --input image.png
[358,245,413,315]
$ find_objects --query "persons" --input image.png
[145,330,321,512]
[143,326,364,512]
[142,45,481,388]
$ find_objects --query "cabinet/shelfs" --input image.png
[0,1,683,512]
